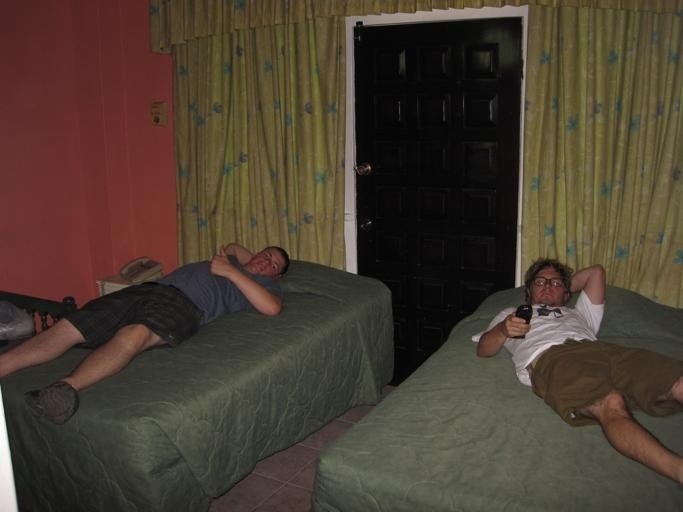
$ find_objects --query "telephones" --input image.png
[120,256,162,283]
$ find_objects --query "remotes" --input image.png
[514,304,533,339]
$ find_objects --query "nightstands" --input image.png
[94,275,165,297]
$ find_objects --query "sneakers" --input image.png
[24,382,79,425]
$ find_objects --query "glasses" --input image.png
[533,276,564,287]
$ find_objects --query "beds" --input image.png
[304,278,683,512]
[1,257,397,512]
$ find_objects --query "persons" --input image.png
[0,242,289,424]
[468,257,683,487]
